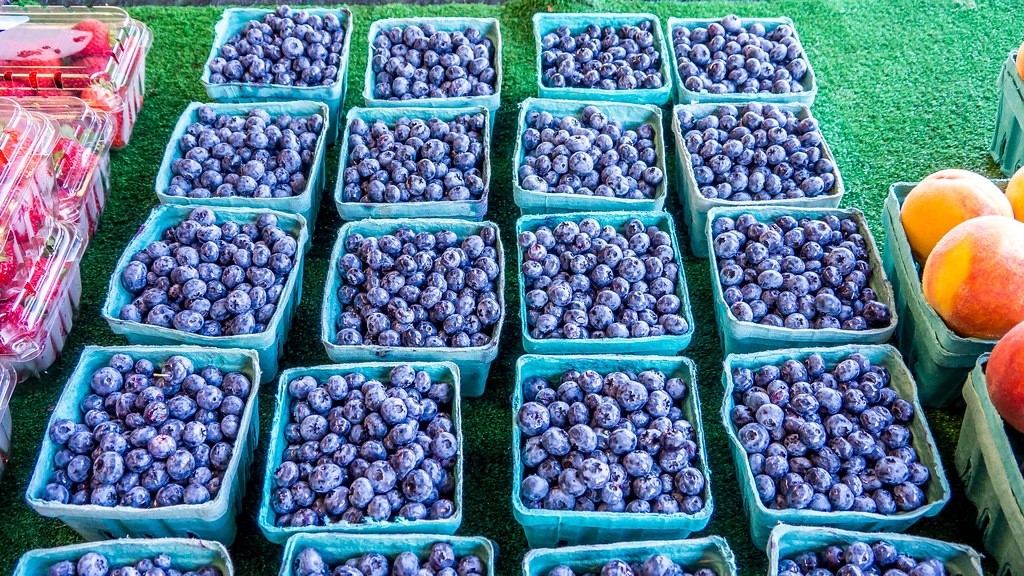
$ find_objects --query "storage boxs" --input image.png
[0,4,1023,575]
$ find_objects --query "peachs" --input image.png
[884,165,1024,436]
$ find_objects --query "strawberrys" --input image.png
[0,9,150,457]
